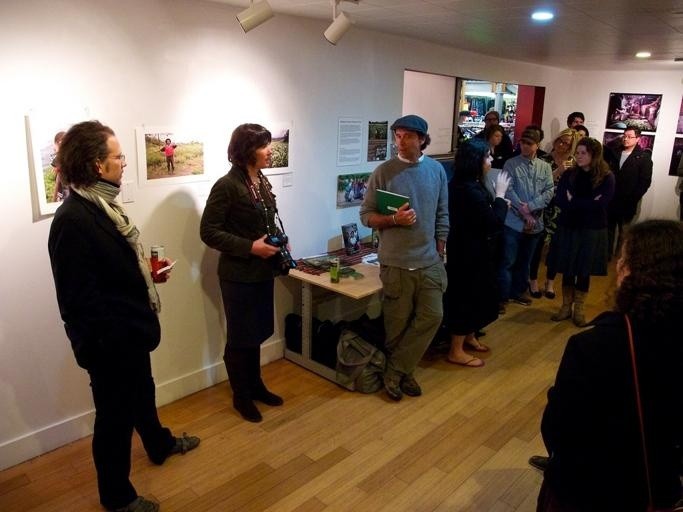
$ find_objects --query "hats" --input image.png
[390,115,427,133]
[519,130,540,145]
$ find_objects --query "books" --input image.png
[375,185,416,217]
[483,167,511,203]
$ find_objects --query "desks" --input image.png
[281,246,382,392]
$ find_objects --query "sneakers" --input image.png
[383,368,403,400]
[400,372,421,396]
[513,296,531,307]
[528,456,549,471]
[168,432,201,455]
[102,495,160,511]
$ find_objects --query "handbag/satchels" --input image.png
[335,330,385,393]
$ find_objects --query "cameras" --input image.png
[264,233,297,277]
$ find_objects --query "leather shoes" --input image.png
[256,391,283,406]
[528,281,542,298]
[545,281,555,299]
[233,398,262,422]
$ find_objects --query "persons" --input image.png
[48,134,68,200]
[528,454,551,474]
[609,96,657,131]
[348,230,358,249]
[156,135,180,172]
[357,115,453,400]
[447,134,512,370]
[197,122,292,421]
[536,218,682,511]
[551,138,617,330]
[51,123,200,511]
[673,149,682,226]
[499,128,555,316]
[342,178,369,204]
[457,110,654,304]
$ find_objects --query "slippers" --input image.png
[448,355,484,367]
[463,344,488,352]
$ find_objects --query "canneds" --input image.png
[150,245,165,283]
[330,257,340,283]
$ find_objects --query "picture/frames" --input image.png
[239,118,295,177]
[25,114,64,216]
[133,125,207,189]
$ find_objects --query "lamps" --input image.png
[232,1,277,36]
[324,0,353,47]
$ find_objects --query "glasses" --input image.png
[623,136,637,139]
[107,155,125,161]
[484,119,497,121]
[557,138,570,146]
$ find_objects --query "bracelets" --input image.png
[495,194,506,200]
[390,213,398,226]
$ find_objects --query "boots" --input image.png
[551,286,574,320]
[572,290,588,327]
